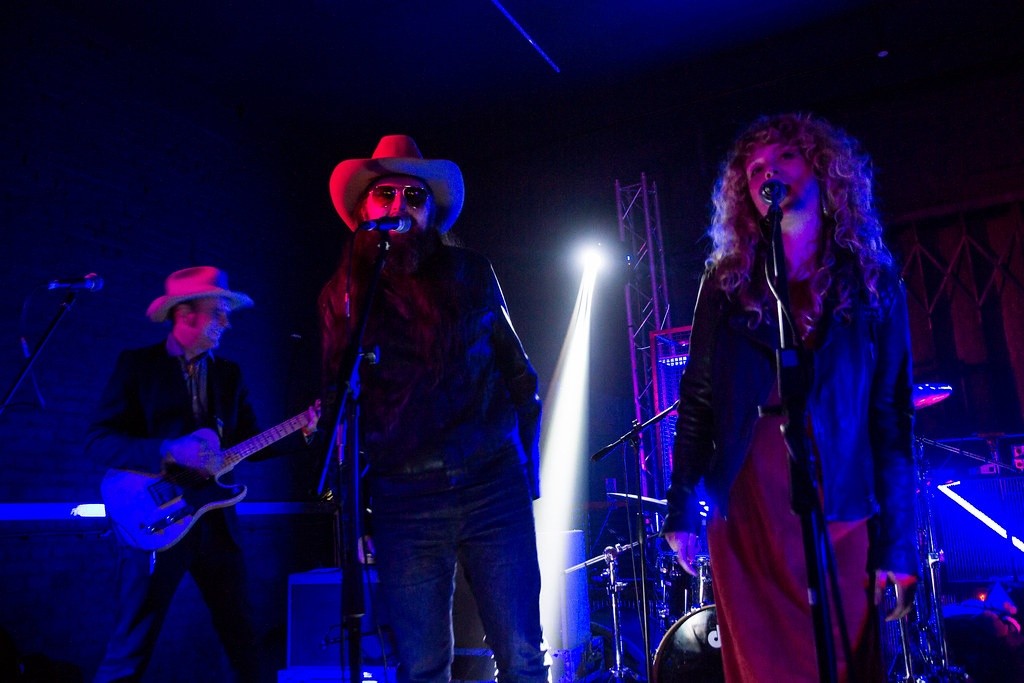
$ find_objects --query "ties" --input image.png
[179,355,205,430]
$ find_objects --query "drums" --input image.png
[651,604,724,682]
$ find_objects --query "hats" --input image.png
[147,266,256,322]
[329,134,465,236]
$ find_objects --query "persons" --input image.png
[668,114,930,683]
[94,265,324,683]
[308,129,552,682]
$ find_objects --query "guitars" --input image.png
[101,394,341,554]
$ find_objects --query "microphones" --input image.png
[642,399,681,428]
[758,179,788,205]
[359,216,412,233]
[46,272,104,293]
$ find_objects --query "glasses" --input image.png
[365,185,434,210]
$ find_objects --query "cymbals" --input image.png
[912,384,951,408]
